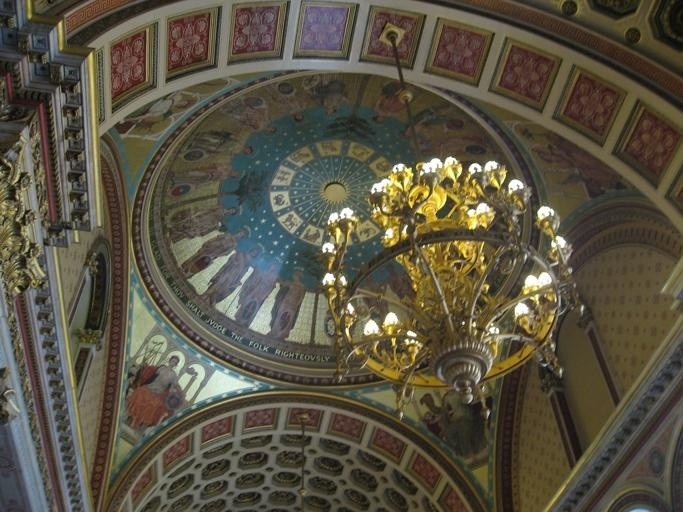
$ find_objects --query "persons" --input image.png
[120,91,196,125]
[125,355,181,429]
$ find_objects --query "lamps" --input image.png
[318,18,588,422]
